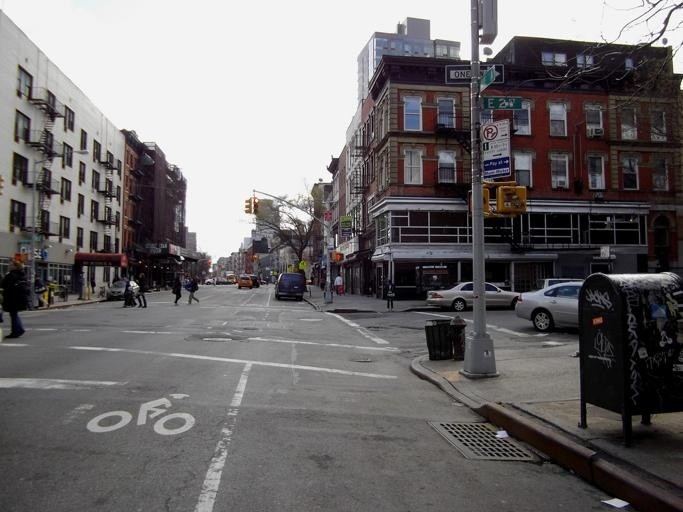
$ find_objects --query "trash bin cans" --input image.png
[425,315,466,361]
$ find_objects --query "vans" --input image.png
[535,277,585,293]
[274,272,305,300]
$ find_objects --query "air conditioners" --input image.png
[589,127,605,136]
[556,179,566,187]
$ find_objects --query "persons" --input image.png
[34,273,49,308]
[320,277,325,291]
[3,259,26,338]
[187,277,199,305]
[91,278,96,293]
[334,274,343,296]
[172,276,181,304]
[386,280,396,312]
[124,281,137,307]
[136,273,149,308]
[78,274,83,297]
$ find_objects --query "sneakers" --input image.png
[137,305,147,308]
[387,308,395,312]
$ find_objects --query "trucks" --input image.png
[222,270,236,285]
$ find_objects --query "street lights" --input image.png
[27,146,90,310]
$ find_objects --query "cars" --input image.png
[237,273,268,290]
[106,278,141,300]
[422,279,523,312]
[514,282,583,334]
[205,276,226,285]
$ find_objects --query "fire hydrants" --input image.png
[449,314,469,364]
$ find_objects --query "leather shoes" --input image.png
[5,328,26,338]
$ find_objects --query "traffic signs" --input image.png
[474,65,499,95]
[445,63,505,85]
[480,94,521,110]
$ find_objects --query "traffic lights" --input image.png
[244,197,253,216]
[253,198,260,215]
[328,250,335,263]
[335,253,345,263]
